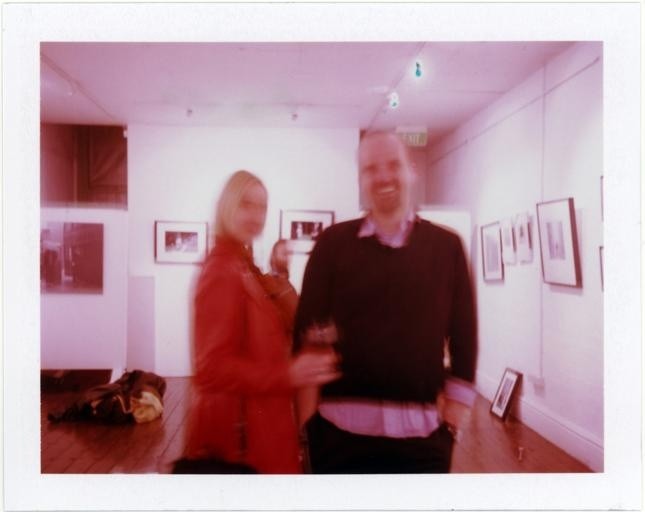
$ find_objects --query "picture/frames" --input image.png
[488,367,523,423]
[279,210,335,254]
[480,222,503,284]
[534,198,584,291]
[152,218,208,266]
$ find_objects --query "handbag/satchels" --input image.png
[170,442,255,475]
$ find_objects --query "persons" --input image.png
[180,170,343,474]
[290,129,478,474]
[260,239,300,317]
